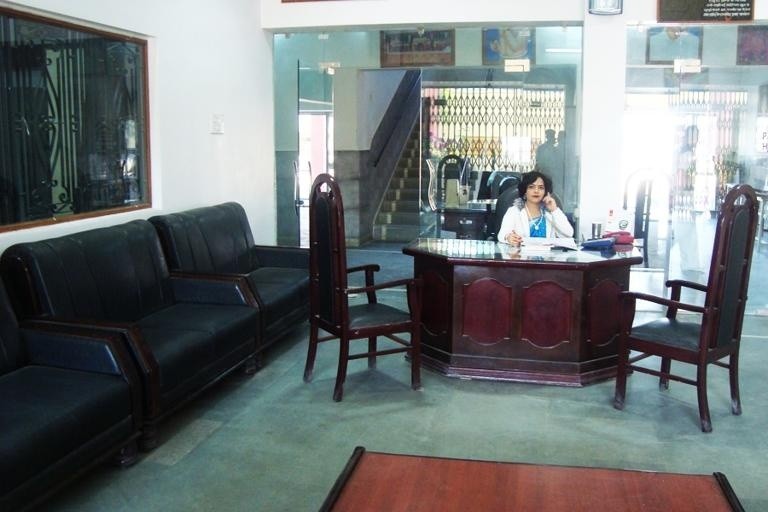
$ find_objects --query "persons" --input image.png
[495,170,574,248]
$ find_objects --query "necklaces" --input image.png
[524,202,543,230]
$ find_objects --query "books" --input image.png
[520,236,578,253]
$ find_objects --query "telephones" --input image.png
[602,230,634,244]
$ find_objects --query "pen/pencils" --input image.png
[512,230,522,243]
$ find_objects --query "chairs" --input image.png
[608,183,760,433]
[303,173,424,403]
